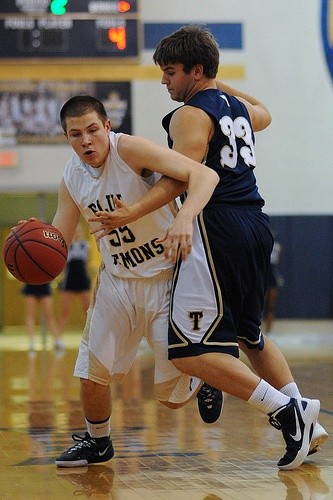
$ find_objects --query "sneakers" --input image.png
[189,377,224,426]
[55,431,114,467]
[266,397,320,471]
[306,420,329,458]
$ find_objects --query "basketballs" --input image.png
[2,220,68,285]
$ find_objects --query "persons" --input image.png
[11,95,219,466]
[89,28,327,470]
[21,223,295,358]
